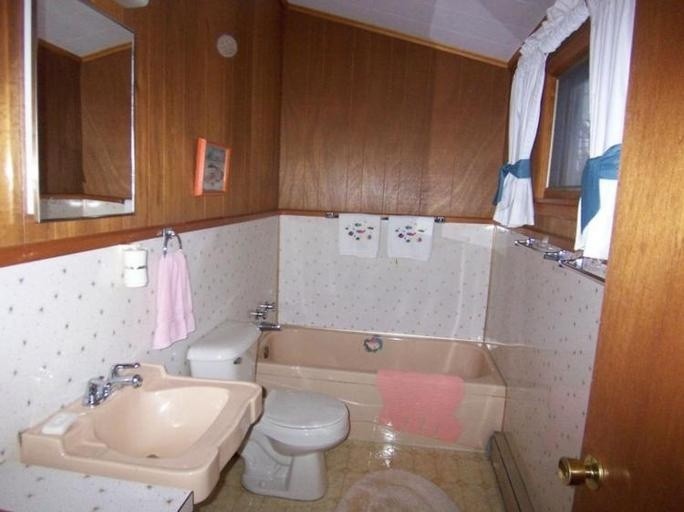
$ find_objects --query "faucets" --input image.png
[81,374,143,409]
[258,323,281,331]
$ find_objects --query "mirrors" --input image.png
[192,136,234,196]
[23,0,137,222]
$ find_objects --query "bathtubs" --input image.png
[256,323,509,454]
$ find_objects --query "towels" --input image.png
[378,368,465,443]
[387,215,434,261]
[155,250,195,349]
[337,213,381,259]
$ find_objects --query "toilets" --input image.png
[185,318,352,502]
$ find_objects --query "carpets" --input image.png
[335,469,459,511]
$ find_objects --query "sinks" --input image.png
[17,361,264,505]
